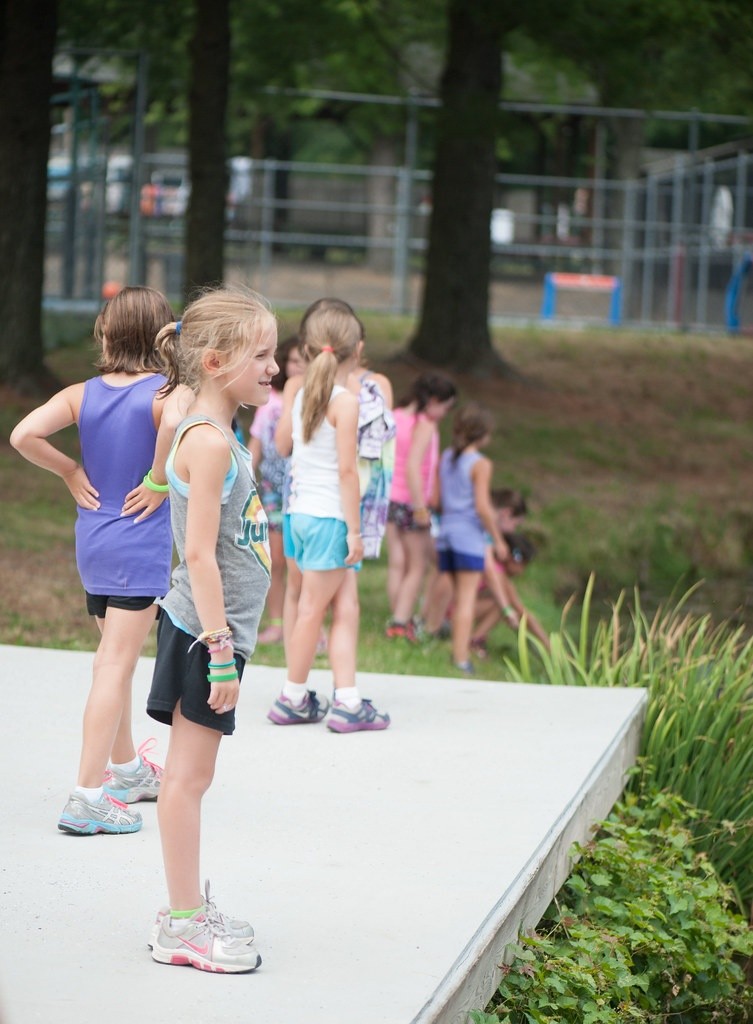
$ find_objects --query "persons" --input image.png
[248,299,552,733]
[10,286,194,835]
[147,289,279,972]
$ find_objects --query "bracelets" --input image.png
[345,534,362,540]
[411,506,427,516]
[186,627,239,681]
[142,470,170,493]
[502,605,513,616]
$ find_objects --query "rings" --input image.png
[223,704,228,712]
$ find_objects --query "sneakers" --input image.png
[267,690,330,725]
[152,909,262,974]
[57,770,142,835]
[327,699,390,733]
[103,738,164,804]
[147,879,254,950]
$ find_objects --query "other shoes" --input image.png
[384,609,487,675]
[314,626,328,655]
[256,619,284,647]
